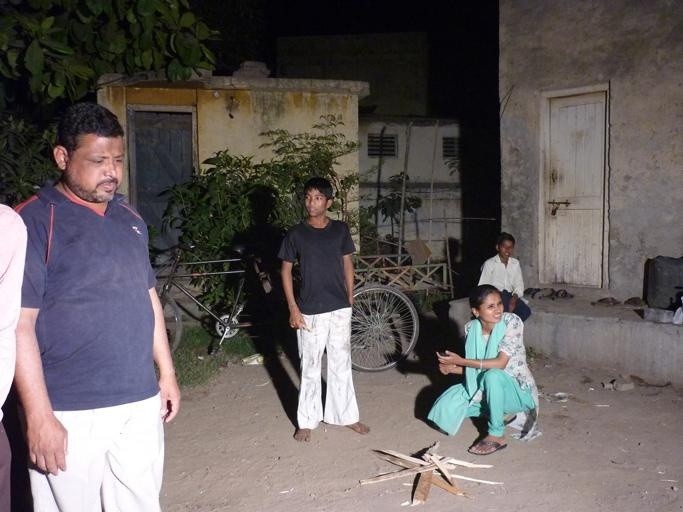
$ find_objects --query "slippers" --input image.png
[524,288,573,299]
[467,439,507,455]
[624,297,647,309]
[592,297,621,307]
[504,414,517,426]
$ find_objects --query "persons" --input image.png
[475,231,533,324]
[277,174,370,443]
[0,202,28,512]
[424,282,543,456]
[11,101,184,512]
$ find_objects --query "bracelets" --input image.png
[479,359,482,370]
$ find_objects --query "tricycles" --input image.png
[157,240,456,375]
